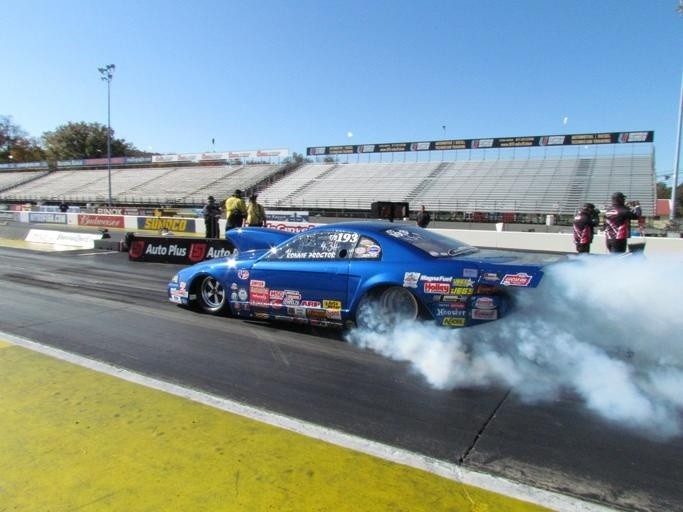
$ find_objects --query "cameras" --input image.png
[630,201,636,206]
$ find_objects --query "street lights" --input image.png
[98,64,115,208]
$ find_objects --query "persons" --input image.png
[59,201,68,211]
[242,193,267,228]
[603,192,641,252]
[572,203,599,252]
[161,227,174,236]
[102,228,110,239]
[202,196,221,239]
[225,190,247,231]
[416,205,430,228]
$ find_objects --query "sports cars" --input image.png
[167,220,569,347]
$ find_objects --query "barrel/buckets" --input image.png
[546,215,554,226]
[629,243,645,253]
